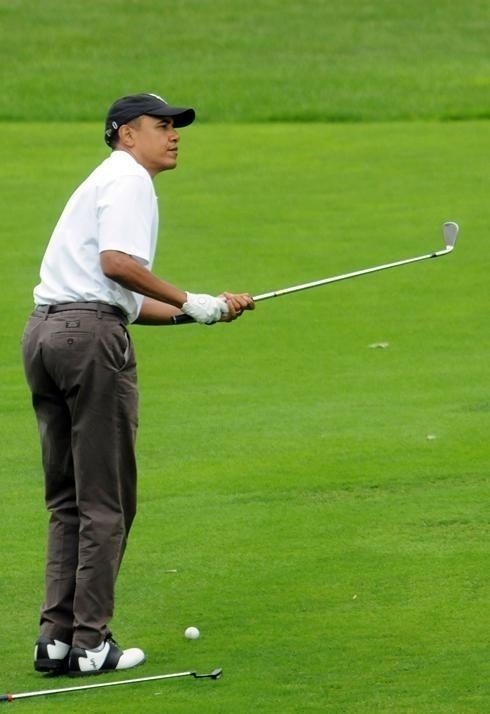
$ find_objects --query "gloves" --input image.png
[181,291,228,324]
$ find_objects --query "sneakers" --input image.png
[34,637,146,677]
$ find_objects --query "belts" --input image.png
[36,302,129,326]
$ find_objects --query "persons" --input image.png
[24,94,255,676]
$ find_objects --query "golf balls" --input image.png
[183,626,202,641]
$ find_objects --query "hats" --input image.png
[105,93,195,146]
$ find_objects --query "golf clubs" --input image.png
[170,222,458,323]
[1,668,224,702]
